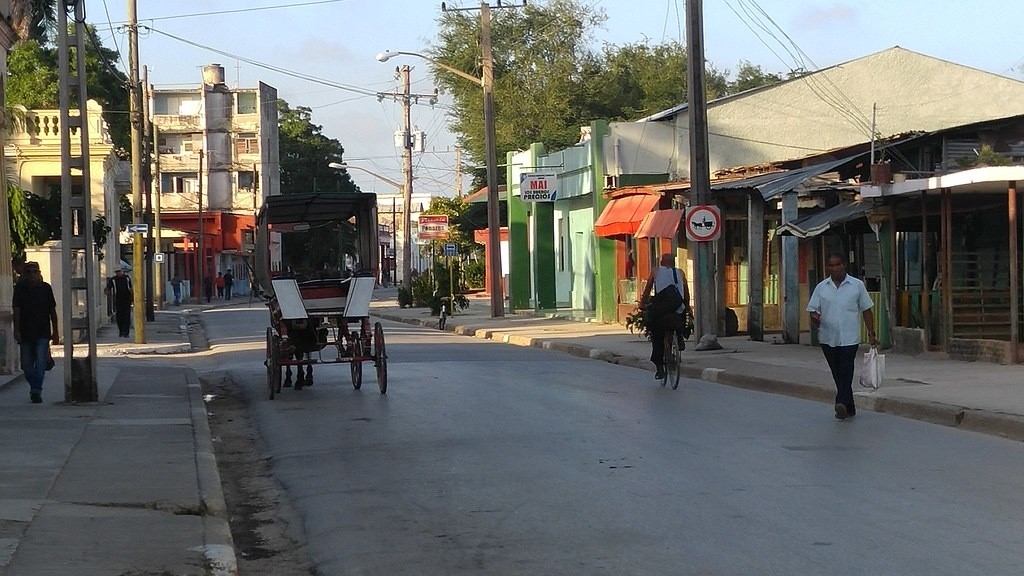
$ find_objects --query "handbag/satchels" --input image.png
[650,284,683,315]
[859,348,886,390]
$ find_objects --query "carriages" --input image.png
[255,193,388,400]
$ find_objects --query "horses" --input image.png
[275,325,314,391]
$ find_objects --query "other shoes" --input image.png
[834,403,856,419]
[118,333,129,338]
[29,392,42,403]
[655,372,665,379]
[678,336,685,351]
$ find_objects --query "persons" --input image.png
[806,254,879,420]
[638,253,690,380]
[170,273,185,306]
[104,268,134,338]
[224,269,233,300]
[203,272,214,303]
[214,272,223,299]
[12,264,60,403]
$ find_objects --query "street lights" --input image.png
[376,50,504,318]
[329,162,412,306]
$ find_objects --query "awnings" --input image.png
[594,193,684,238]
[768,201,883,241]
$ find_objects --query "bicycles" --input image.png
[433,290,455,330]
[641,296,681,389]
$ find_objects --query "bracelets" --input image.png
[868,332,876,337]
[53,331,58,336]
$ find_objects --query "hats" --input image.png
[114,265,122,272]
[227,269,232,271]
